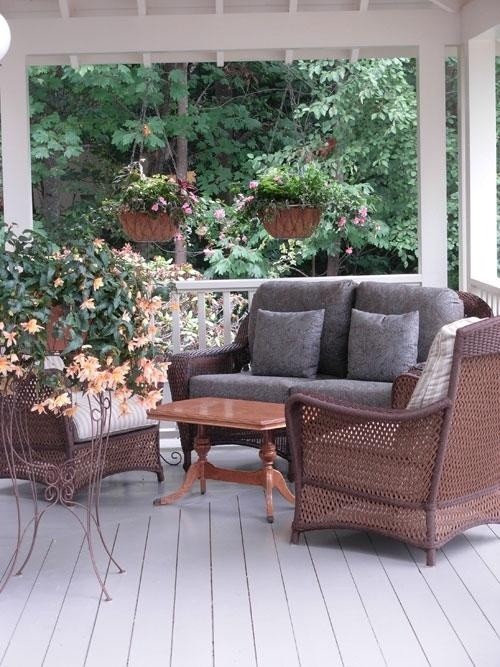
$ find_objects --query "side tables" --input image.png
[0,350,126,601]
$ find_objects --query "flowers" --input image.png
[0,222,172,419]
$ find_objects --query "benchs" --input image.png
[168,291,494,472]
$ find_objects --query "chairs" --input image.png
[0,345,165,506]
[284,315,500,567]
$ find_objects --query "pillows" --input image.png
[247,279,358,378]
[347,308,420,382]
[404,316,490,411]
[353,281,465,363]
[0,345,74,401]
[251,308,326,379]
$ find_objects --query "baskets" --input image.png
[258,205,320,239]
[119,211,181,243]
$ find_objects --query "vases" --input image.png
[46,300,92,350]
